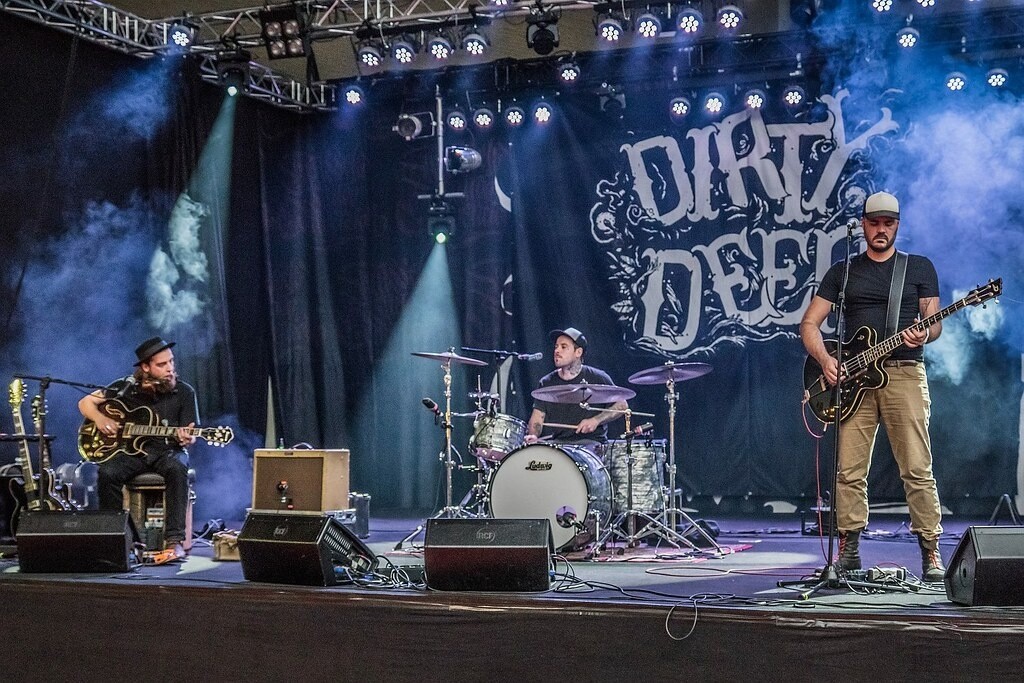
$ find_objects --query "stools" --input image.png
[127,469,196,550]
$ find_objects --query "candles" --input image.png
[669,80,806,116]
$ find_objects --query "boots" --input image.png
[918,537,947,581]
[813,529,862,573]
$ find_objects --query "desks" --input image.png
[250,447,351,514]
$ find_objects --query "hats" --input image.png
[549,327,588,353]
[863,191,901,220]
[132,336,177,367]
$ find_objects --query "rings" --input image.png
[104,424,114,432]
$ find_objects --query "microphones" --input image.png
[117,375,136,398]
[846,217,859,229]
[422,397,446,419]
[619,422,653,440]
[519,352,543,361]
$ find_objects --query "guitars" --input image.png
[76,399,235,464]
[9,377,70,542]
[29,393,87,514]
[802,278,1003,423]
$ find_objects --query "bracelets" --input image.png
[922,327,930,346]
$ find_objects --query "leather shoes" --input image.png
[165,541,186,559]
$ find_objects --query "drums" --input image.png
[604,438,668,514]
[488,442,614,553]
[467,412,528,461]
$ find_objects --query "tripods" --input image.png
[580,404,702,561]
[776,226,912,600]
[392,376,478,550]
[614,383,724,556]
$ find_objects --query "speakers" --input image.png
[16,509,143,572]
[943,524,1024,606]
[236,448,379,586]
[423,517,558,591]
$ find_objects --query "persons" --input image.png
[526,327,630,457]
[797,190,948,583]
[78,337,201,563]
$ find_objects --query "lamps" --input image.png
[447,97,553,131]
[392,112,423,141]
[868,0,937,51]
[986,64,1009,89]
[596,1,746,44]
[945,66,969,94]
[393,35,418,66]
[443,146,482,171]
[557,58,579,83]
[462,25,489,55]
[532,28,556,56]
[428,29,457,60]
[359,38,385,69]
[344,81,373,105]
[224,73,242,99]
[169,20,195,54]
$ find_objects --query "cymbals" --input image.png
[531,383,637,405]
[628,363,714,385]
[411,351,488,367]
